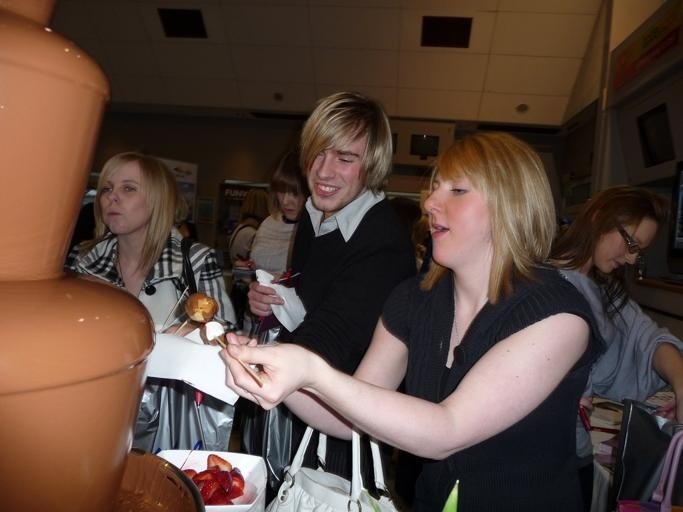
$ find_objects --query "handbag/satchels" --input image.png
[264,465,399,512]
[151,379,236,455]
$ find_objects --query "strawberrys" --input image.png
[177,454,242,506]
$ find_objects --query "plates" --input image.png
[156,449,268,512]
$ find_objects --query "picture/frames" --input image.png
[194,196,216,225]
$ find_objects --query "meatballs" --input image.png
[184,291,217,325]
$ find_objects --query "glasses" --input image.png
[614,220,641,255]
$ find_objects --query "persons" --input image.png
[232,146,308,341]
[228,188,270,264]
[66,148,238,449]
[390,197,422,239]
[551,183,680,512]
[245,89,423,507]
[217,130,608,512]
